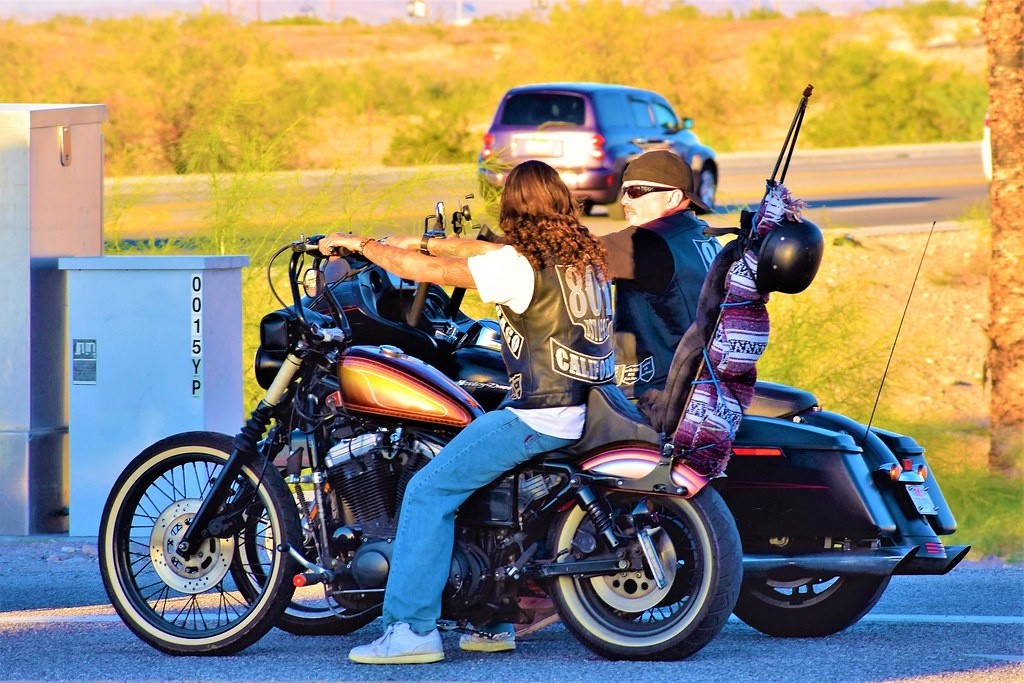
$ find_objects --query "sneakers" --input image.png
[458,622,516,652]
[348,621,445,664]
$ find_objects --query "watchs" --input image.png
[420,233,435,255]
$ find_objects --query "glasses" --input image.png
[622,184,685,199]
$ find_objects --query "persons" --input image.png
[318,160,615,663]
[512,150,723,636]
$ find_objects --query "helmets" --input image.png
[756,212,824,296]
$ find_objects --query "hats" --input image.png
[622,150,710,210]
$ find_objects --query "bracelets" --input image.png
[358,237,376,255]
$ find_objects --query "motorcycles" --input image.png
[98,233,745,662]
[228,191,972,639]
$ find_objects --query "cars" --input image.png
[477,82,719,214]
